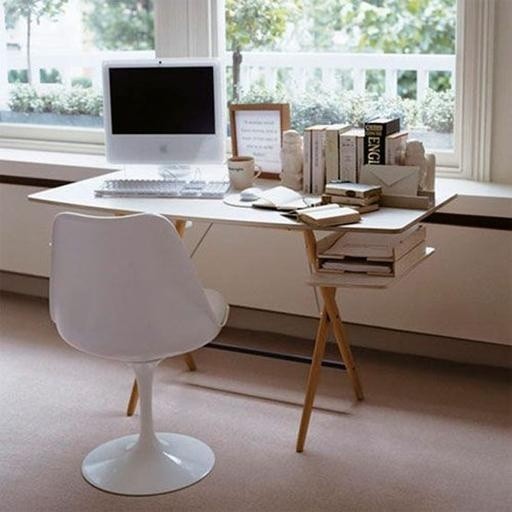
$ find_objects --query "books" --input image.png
[251,117,438,288]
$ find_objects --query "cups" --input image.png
[227,156,261,189]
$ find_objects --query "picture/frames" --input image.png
[229,99,289,182]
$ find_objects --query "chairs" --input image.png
[46,211,231,496]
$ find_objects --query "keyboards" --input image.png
[92,178,233,200]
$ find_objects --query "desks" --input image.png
[26,171,456,451]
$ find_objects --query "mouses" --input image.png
[239,187,262,201]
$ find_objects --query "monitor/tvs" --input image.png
[101,57,226,177]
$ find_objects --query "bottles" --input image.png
[281,128,303,190]
